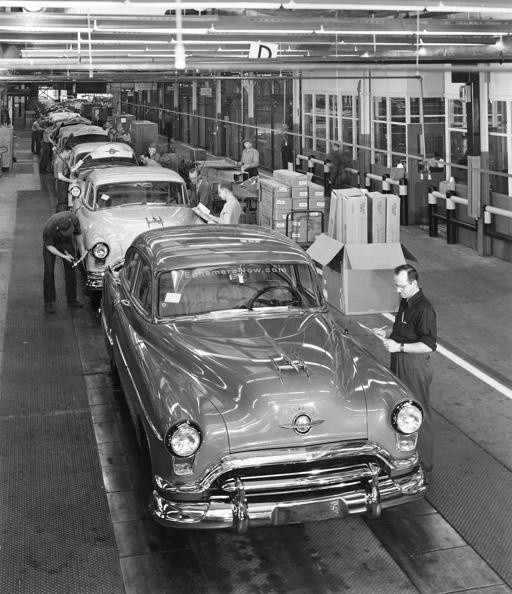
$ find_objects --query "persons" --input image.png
[54,145,76,213]
[139,143,162,166]
[163,109,173,142]
[103,120,131,145]
[234,138,260,181]
[42,211,85,313]
[31,116,60,173]
[372,264,437,484]
[207,182,242,224]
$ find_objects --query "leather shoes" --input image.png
[45,303,56,313]
[68,300,83,308]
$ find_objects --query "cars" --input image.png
[74,168,199,290]
[38,109,142,203]
[99,223,426,531]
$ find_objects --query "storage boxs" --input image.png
[256,169,424,315]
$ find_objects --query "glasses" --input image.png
[392,284,409,291]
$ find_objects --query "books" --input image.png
[192,202,211,222]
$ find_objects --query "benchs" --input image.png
[145,278,294,316]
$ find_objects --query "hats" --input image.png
[242,137,254,143]
[56,216,75,237]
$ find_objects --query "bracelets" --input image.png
[400,343,404,353]
